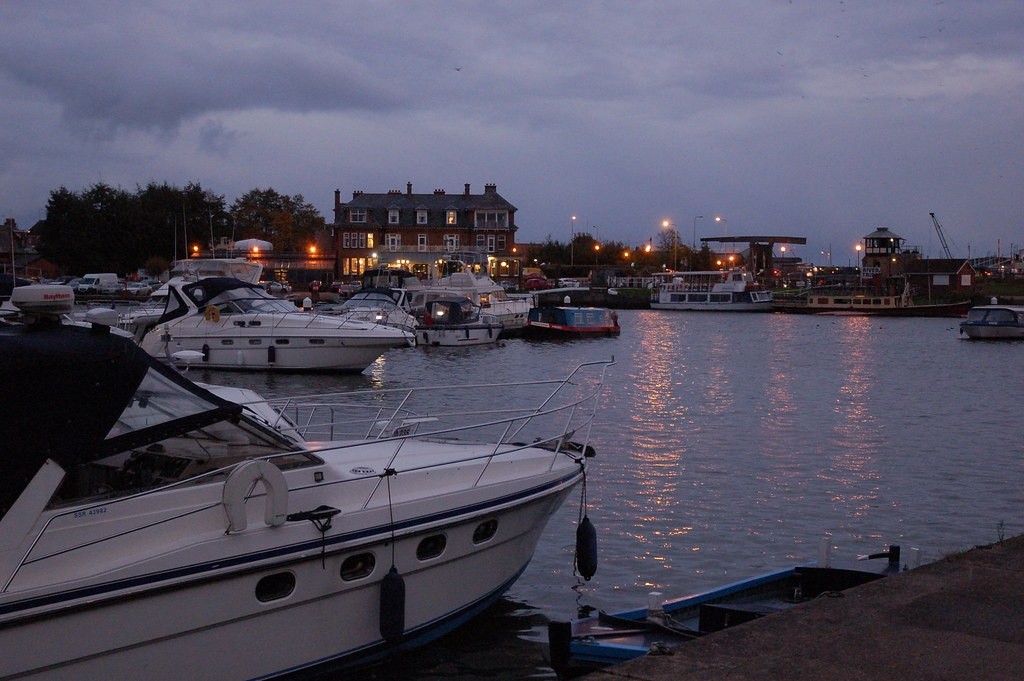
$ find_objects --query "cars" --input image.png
[123,279,163,296]
[496,275,581,293]
[0,271,77,296]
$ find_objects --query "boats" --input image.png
[959,297,1024,340]
[0,285,618,681]
[529,295,621,335]
[651,267,773,312]
[772,272,974,316]
[540,535,920,681]
[116,205,415,373]
[318,254,533,347]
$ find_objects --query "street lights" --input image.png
[593,225,599,265]
[663,221,678,271]
[716,217,727,270]
[780,247,785,269]
[571,216,577,266]
[693,216,704,249]
[856,245,861,271]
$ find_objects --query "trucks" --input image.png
[78,272,122,294]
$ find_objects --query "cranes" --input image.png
[928,211,964,259]
